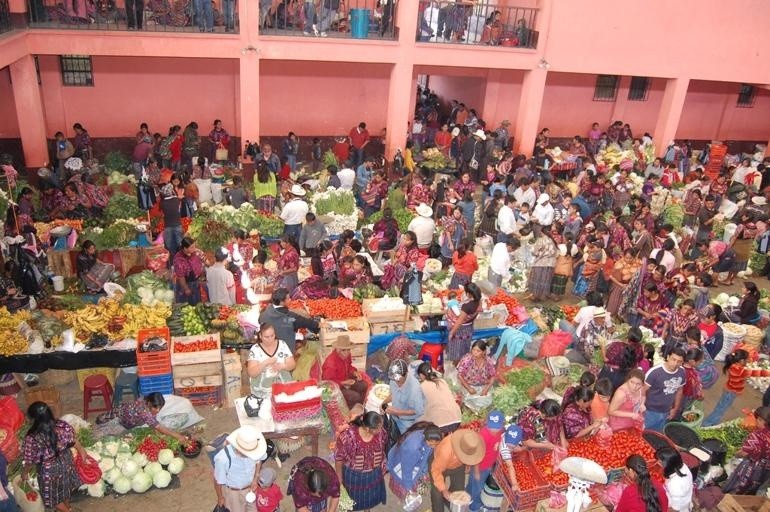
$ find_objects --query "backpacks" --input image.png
[203,432,230,468]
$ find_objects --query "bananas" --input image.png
[0,293,172,356]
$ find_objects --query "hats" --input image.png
[225,423,279,488]
[450,117,511,159]
[591,307,611,319]
[536,193,550,204]
[286,183,307,197]
[331,335,356,351]
[2,154,84,248]
[412,202,435,219]
[503,424,526,447]
[485,410,507,430]
[449,427,487,467]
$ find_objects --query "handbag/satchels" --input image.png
[215,143,229,162]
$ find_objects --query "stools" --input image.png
[83,374,114,420]
[115,373,139,406]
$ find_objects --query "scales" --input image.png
[134,224,151,247]
[49,226,71,250]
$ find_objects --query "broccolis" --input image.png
[200,201,259,243]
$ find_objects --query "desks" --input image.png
[548,170,571,181]
[237,394,324,457]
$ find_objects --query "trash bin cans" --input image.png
[350,8,371,39]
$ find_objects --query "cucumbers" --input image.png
[165,301,224,334]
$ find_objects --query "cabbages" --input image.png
[79,440,185,498]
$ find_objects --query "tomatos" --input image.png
[153,217,192,233]
[173,336,218,408]
[261,295,363,335]
[435,286,522,326]
[503,427,655,495]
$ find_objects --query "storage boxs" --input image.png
[709,507,720,512]
[716,493,770,512]
[168,296,500,411]
[535,491,610,512]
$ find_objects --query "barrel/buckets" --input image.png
[52,275,64,291]
[351,7,369,38]
